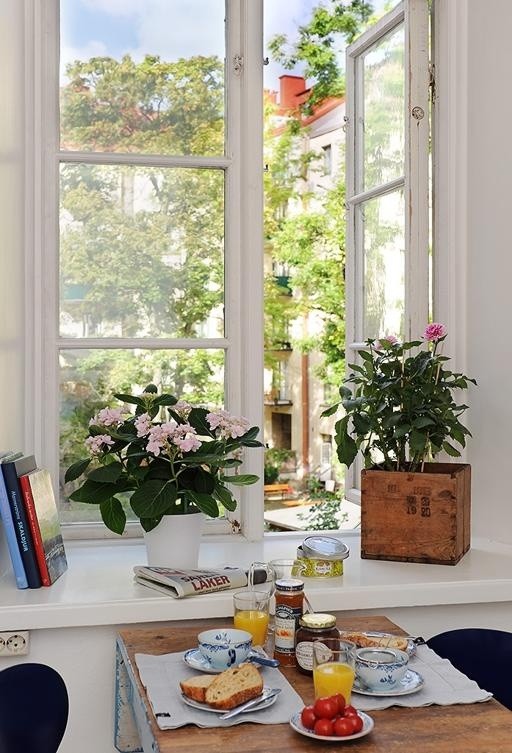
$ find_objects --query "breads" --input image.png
[204,663,263,710]
[341,630,411,652]
[179,674,215,702]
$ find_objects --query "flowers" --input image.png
[320,320,477,472]
[61,382,265,536]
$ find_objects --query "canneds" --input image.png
[293,611,342,676]
[291,534,350,578]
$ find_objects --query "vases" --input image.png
[359,460,473,566]
[144,511,208,569]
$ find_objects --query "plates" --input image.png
[180,688,277,713]
[289,710,374,741]
[183,648,266,673]
[351,668,425,696]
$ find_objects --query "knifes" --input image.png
[220,689,282,720]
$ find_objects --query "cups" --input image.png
[312,638,356,706]
[233,590,271,648]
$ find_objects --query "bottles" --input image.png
[248,558,313,634]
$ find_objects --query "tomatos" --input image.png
[301,691,364,737]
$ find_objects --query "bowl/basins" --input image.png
[355,647,410,690]
[197,628,253,667]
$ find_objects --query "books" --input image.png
[0,450,68,589]
